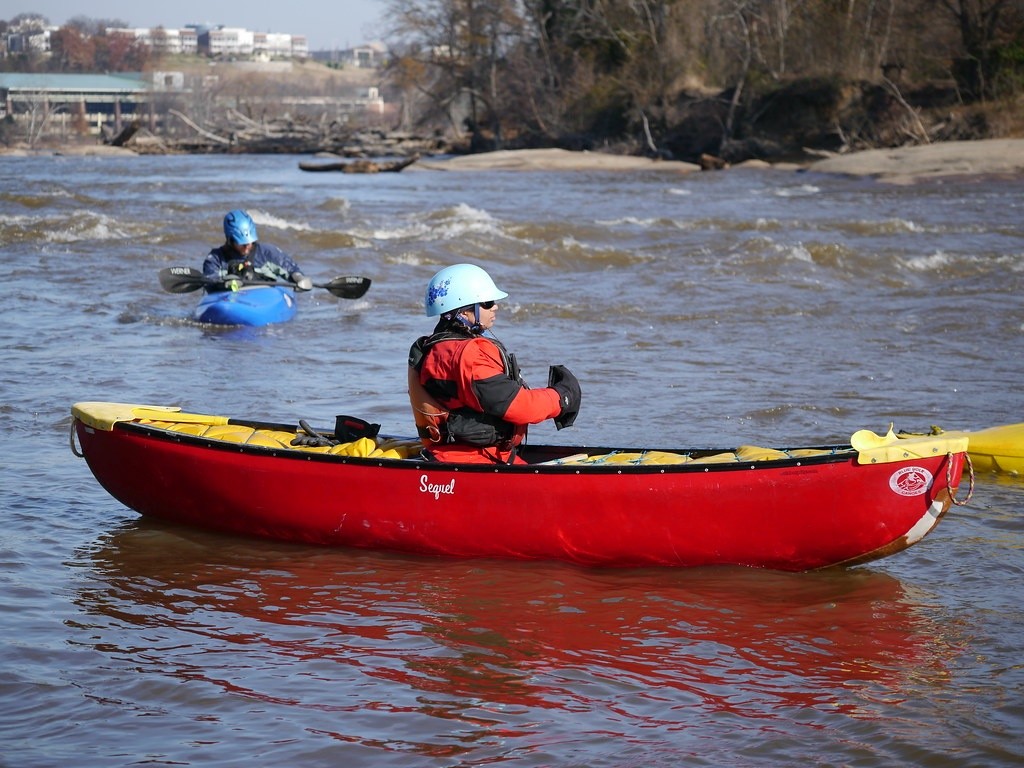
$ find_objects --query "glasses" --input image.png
[480,301,494,309]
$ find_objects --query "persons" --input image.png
[203,209,313,295]
[409,263,581,467]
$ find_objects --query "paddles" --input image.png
[157,266,372,299]
[131,408,419,440]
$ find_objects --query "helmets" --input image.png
[223,209,258,245]
[335,415,381,443]
[425,264,508,317]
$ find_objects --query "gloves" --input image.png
[547,364,581,431]
[289,272,313,291]
[221,274,243,290]
[290,420,334,446]
